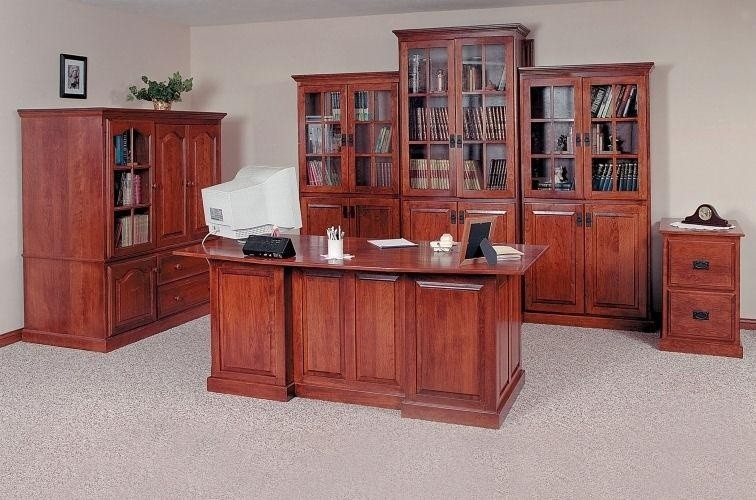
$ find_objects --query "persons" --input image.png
[69,66,81,89]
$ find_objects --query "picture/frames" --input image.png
[59,54,89,100]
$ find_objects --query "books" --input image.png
[592,122,605,154]
[376,162,393,186]
[133,214,149,244]
[325,124,340,153]
[374,92,390,120]
[429,106,448,140]
[617,160,638,191]
[463,159,482,190]
[486,159,507,189]
[117,172,132,205]
[373,125,392,152]
[463,64,481,91]
[431,159,449,190]
[591,86,612,118]
[592,160,613,190]
[325,157,340,185]
[463,107,483,140]
[355,125,370,153]
[306,124,322,154]
[409,158,428,189]
[115,216,131,247]
[486,106,506,140]
[114,130,130,165]
[306,160,322,186]
[357,158,370,185]
[354,91,368,122]
[408,107,426,140]
[324,91,340,122]
[305,114,322,121]
[133,173,141,204]
[497,62,506,91]
[616,84,638,117]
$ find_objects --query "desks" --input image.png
[173,230,550,431]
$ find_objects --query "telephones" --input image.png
[429,232,460,252]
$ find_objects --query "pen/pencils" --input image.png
[326,225,345,240]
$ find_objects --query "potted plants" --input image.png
[126,70,194,110]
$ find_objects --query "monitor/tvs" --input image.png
[200,163,305,243]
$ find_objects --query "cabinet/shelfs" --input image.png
[14,107,229,355]
[292,72,402,239]
[656,219,746,358]
[517,59,655,334]
[391,21,532,245]
